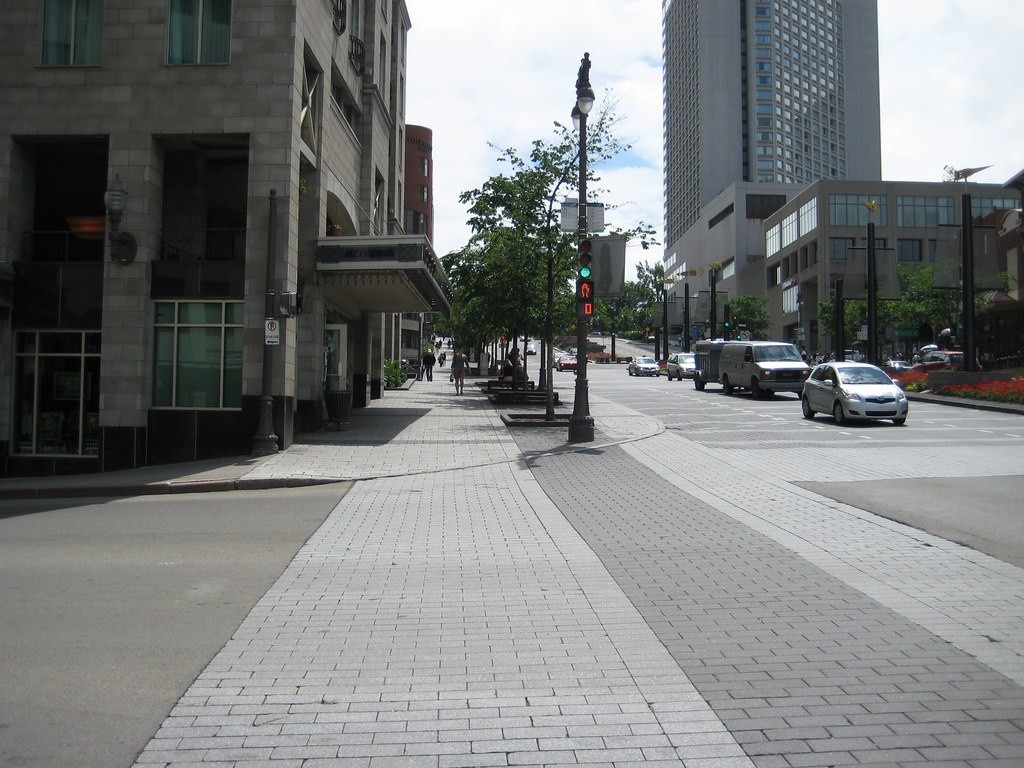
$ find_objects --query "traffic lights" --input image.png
[576,278,595,317]
[577,239,592,278]
[612,322,615,336]
[724,304,730,340]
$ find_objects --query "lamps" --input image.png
[61,203,105,241]
[279,292,298,318]
[296,294,304,315]
[104,172,137,266]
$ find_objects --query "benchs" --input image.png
[488,380,535,390]
[498,391,558,402]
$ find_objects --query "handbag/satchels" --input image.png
[450,373,454,382]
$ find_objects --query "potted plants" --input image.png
[328,224,343,236]
[299,177,312,202]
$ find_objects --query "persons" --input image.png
[423,351,436,381]
[807,350,834,366]
[488,352,490,366]
[439,339,442,347]
[432,335,436,340]
[883,350,904,363]
[678,336,682,345]
[448,339,451,347]
[435,342,439,353]
[912,345,917,357]
[801,345,806,363]
[501,347,529,388]
[452,346,472,396]
[438,352,446,368]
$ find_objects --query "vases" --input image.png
[324,390,353,422]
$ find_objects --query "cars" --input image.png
[552,352,567,367]
[665,354,696,382]
[526,342,537,355]
[628,356,661,377]
[883,360,913,370]
[568,348,588,364]
[556,356,578,372]
[910,351,981,374]
[521,334,532,342]
[802,362,909,425]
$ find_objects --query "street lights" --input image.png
[680,269,696,354]
[704,260,722,340]
[861,198,883,365]
[943,164,993,374]
[559,51,600,443]
[656,279,675,364]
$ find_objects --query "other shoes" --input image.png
[461,392,463,395]
[457,393,460,396]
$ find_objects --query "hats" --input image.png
[428,349,431,352]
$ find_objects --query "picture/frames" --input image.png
[53,372,91,401]
[83,412,102,433]
[28,411,63,440]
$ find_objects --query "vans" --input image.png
[831,350,860,360]
[719,341,811,401]
